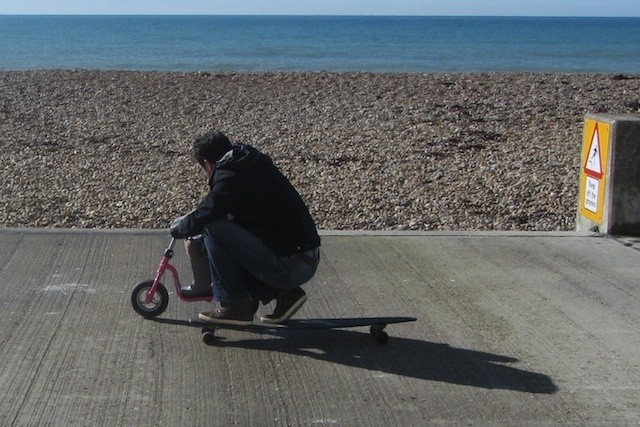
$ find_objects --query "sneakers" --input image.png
[181,285,212,301]
[199,300,254,325]
[261,287,307,323]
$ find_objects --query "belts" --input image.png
[304,248,320,259]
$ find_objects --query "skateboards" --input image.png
[188,316,418,346]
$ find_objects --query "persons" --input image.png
[170,131,320,324]
